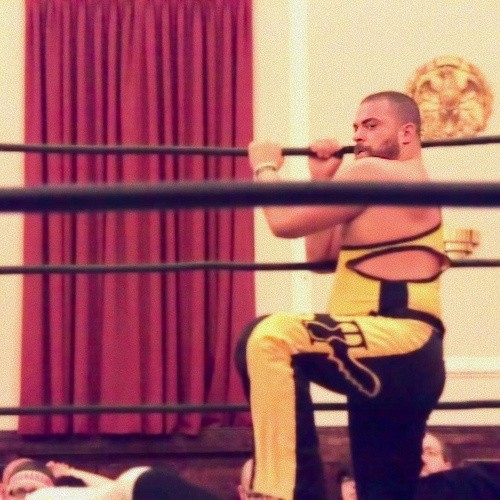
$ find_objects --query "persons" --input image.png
[337,468,371,500]
[236,455,294,500]
[234,91,445,500]
[418,426,464,478]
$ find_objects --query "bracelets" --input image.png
[255,162,278,178]
